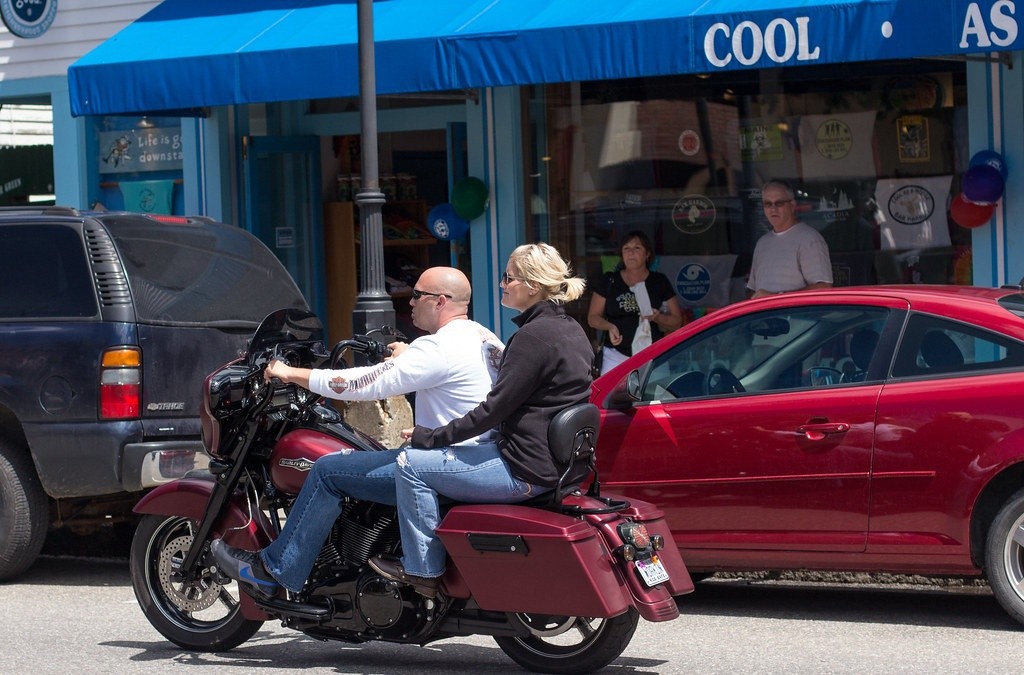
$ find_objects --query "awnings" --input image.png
[69,0,1024,117]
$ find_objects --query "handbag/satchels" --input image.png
[589,350,602,379]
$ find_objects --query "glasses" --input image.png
[761,197,794,209]
[501,272,532,286]
[410,287,452,300]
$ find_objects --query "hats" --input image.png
[963,150,1007,203]
[951,192,996,229]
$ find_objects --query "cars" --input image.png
[593,285,1024,632]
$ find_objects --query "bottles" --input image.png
[659,301,671,314]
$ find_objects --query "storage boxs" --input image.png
[433,488,695,619]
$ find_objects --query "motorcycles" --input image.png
[129,306,697,674]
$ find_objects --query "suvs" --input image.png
[0,207,325,581]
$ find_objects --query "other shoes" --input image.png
[368,555,438,599]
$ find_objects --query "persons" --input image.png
[210,265,504,598]
[366,242,596,600]
[748,179,833,387]
[587,231,683,399]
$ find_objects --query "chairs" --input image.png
[850,328,881,373]
[921,331,967,374]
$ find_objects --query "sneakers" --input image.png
[210,539,283,601]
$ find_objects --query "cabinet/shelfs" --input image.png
[323,197,438,367]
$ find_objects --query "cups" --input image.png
[693,308,716,318]
